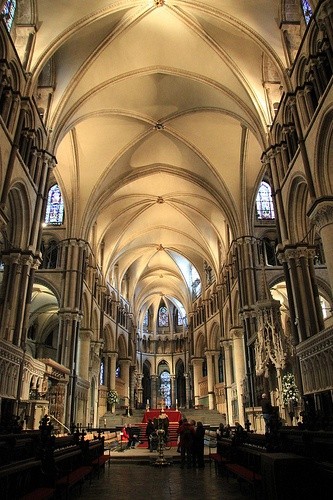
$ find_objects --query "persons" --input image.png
[176,418,196,468]
[146,417,170,450]
[122,423,144,449]
[194,421,205,469]
[235,422,243,432]
[216,423,231,440]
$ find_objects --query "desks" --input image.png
[142,408,182,423]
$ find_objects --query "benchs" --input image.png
[210,454,268,500]
[20,450,111,500]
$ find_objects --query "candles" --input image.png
[147,399,149,404]
[175,399,177,404]
[104,419,106,424]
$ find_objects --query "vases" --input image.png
[292,408,298,426]
[111,403,115,414]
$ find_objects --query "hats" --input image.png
[262,403,272,414]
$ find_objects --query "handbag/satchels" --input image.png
[266,434,283,452]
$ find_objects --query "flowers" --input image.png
[284,371,302,407]
[108,390,120,404]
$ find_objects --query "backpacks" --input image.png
[181,430,193,449]
